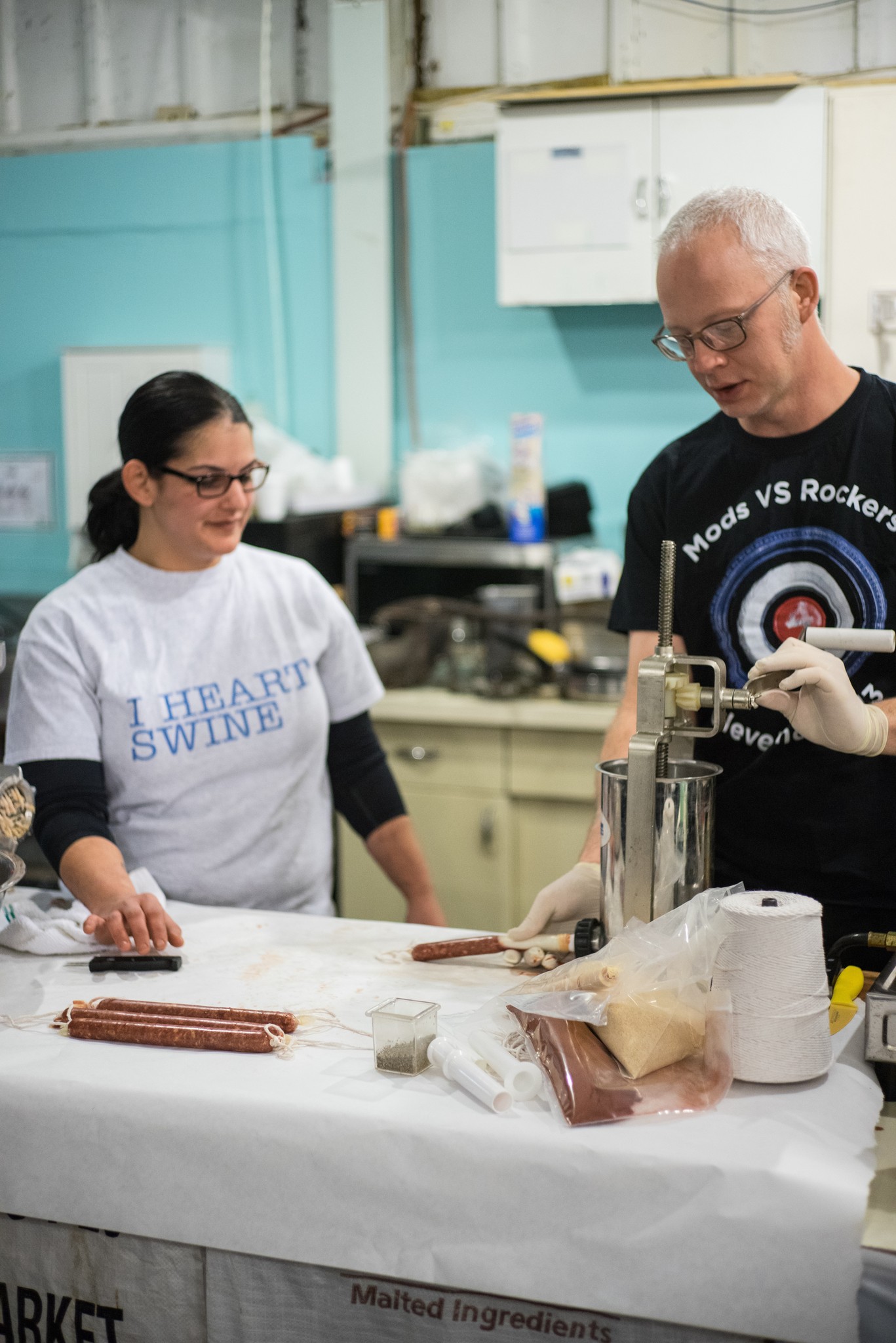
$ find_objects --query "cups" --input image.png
[367,997,440,1076]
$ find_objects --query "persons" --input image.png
[504,188,895,1002]
[2,371,446,956]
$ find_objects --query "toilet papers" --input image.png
[716,885,834,1085]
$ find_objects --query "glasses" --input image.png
[653,269,798,363]
[143,454,270,499]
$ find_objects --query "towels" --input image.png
[0,865,169,956]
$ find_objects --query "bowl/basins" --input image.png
[552,654,627,701]
[1,848,26,908]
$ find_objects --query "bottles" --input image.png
[505,412,546,542]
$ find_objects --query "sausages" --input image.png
[411,935,503,961]
[52,997,298,1053]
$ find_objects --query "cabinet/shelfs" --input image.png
[492,80,895,382]
[336,686,608,931]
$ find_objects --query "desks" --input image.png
[0,887,882,1342]
